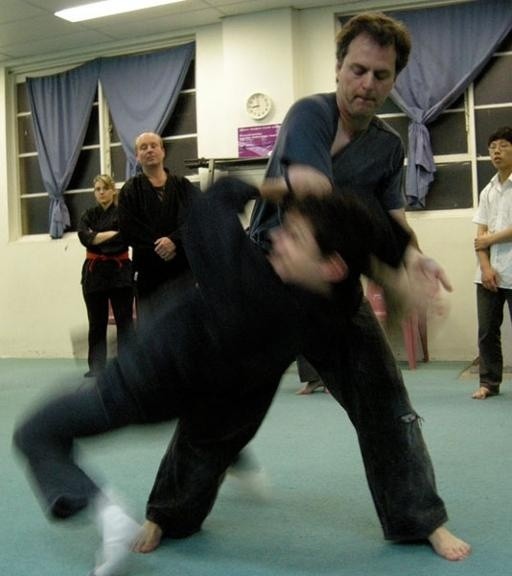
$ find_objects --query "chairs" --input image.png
[365,279,430,370]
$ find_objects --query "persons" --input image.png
[297,358,324,395]
[119,133,194,329]
[131,10,472,561]
[11,162,438,575]
[471,125,512,400]
[77,173,135,377]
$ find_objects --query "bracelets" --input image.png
[283,161,294,194]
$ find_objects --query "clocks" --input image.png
[246,92,271,120]
